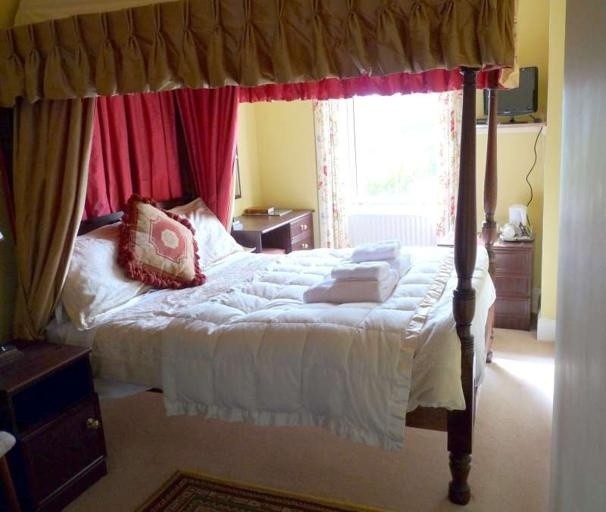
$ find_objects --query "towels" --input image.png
[300,238,416,306]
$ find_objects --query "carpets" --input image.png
[129,466,398,510]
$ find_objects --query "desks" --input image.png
[435,231,536,333]
[231,207,316,253]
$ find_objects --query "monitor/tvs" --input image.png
[484,67,538,124]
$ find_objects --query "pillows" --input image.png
[62,219,157,328]
[116,192,208,289]
[163,194,258,269]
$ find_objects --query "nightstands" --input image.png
[0,342,108,511]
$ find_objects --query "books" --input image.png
[242,209,293,216]
[244,207,275,213]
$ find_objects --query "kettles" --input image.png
[509,204,529,226]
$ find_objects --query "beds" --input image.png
[0,1,519,504]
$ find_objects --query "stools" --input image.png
[261,246,286,253]
